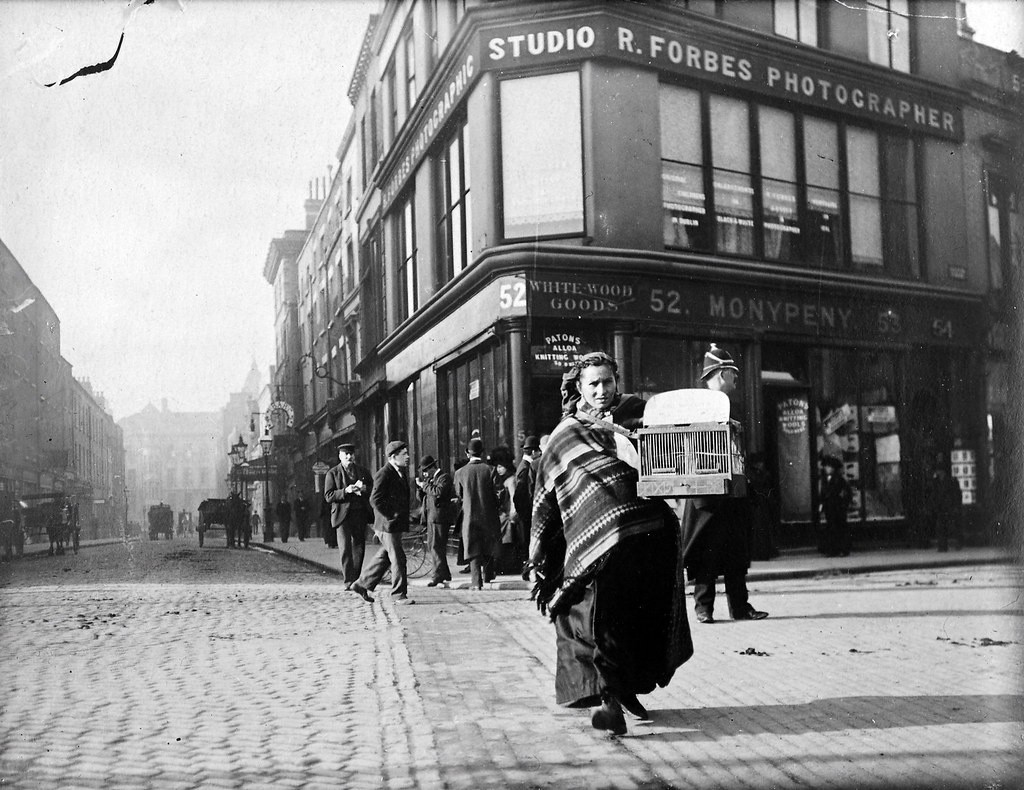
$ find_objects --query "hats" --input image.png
[522,436,537,452]
[417,454,438,471]
[700,343,739,380]
[337,439,355,449]
[821,455,843,469]
[386,441,408,456]
[464,439,484,456]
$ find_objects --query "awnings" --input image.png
[228,454,282,481]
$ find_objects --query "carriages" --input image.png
[176,512,194,537]
[196,491,253,550]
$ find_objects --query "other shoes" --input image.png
[729,604,768,619]
[351,583,374,603]
[344,585,351,591]
[459,565,471,573]
[617,693,649,720]
[591,690,627,735]
[392,597,415,604]
[470,586,481,591]
[483,574,496,583]
[427,573,452,587]
[697,611,714,623]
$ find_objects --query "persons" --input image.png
[932,459,965,550]
[819,456,853,557]
[681,347,770,623]
[249,435,555,603]
[525,352,691,737]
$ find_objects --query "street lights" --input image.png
[228,435,249,502]
[123,487,129,539]
[260,428,274,541]
